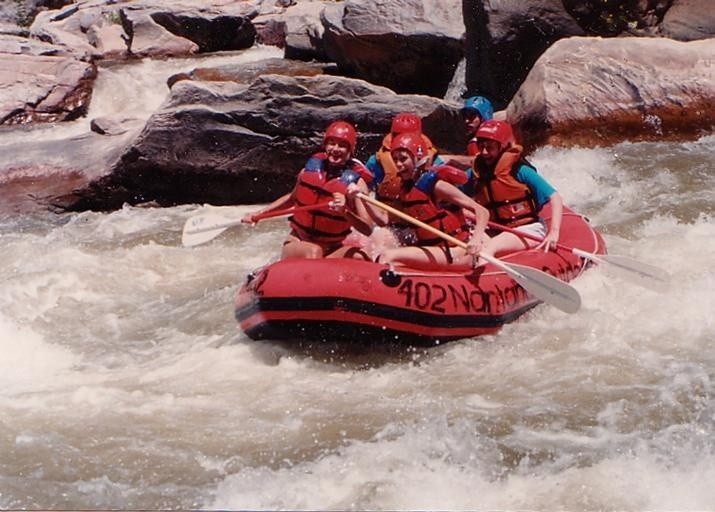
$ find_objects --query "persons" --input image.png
[447,94,494,171]
[242,120,375,264]
[454,118,564,259]
[365,112,446,230]
[346,131,497,273]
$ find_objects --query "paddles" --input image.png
[181,202,335,247]
[464,209,670,295]
[355,192,581,314]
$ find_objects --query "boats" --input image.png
[228,191,606,350]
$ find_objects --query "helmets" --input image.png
[459,95,494,121]
[475,119,513,150]
[390,132,427,159]
[391,113,422,134]
[323,120,356,154]
